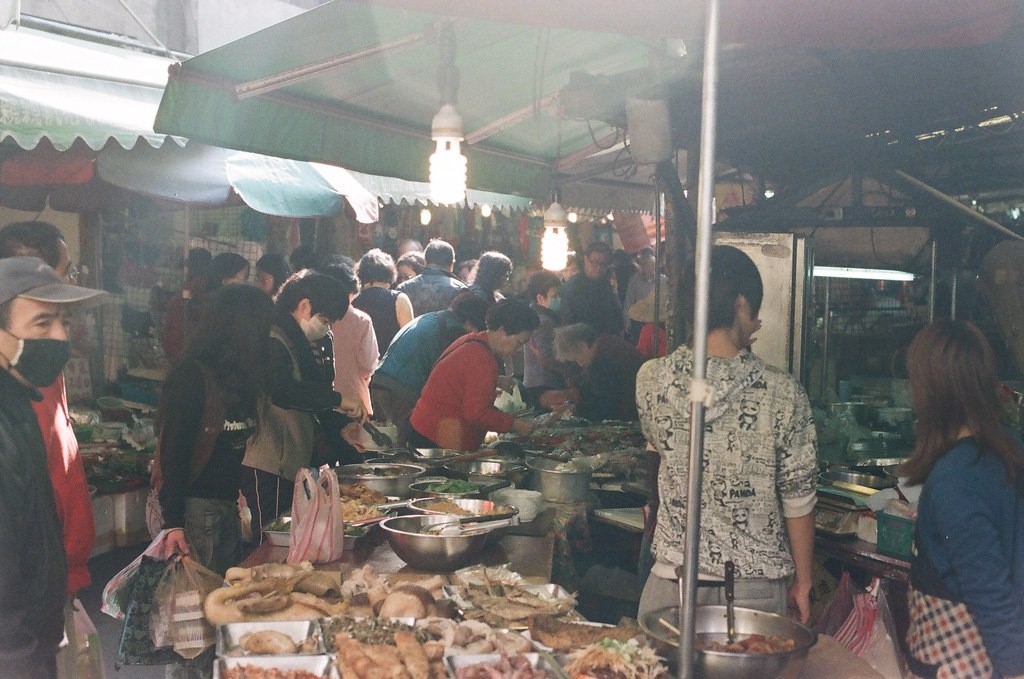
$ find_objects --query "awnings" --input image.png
[345,169,533,215]
[1,14,185,151]
[152,1,1024,213]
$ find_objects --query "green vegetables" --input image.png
[423,477,482,493]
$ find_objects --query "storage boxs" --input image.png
[89,488,152,558]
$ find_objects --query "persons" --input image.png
[406,297,539,449]
[633,244,818,624]
[145,281,274,679]
[0,220,96,679]
[889,314,1024,679]
[146,236,667,547]
[0,255,112,679]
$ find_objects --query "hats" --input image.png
[628,285,669,322]
[0,256,110,310]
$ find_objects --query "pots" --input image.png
[525,457,594,504]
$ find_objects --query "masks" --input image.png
[543,298,562,312]
[300,315,330,342]
[0,328,72,387]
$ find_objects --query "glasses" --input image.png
[253,275,271,285]
[589,257,608,269]
[63,266,80,283]
[397,274,412,281]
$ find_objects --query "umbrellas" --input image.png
[0,140,380,360]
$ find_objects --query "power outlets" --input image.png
[819,208,843,220]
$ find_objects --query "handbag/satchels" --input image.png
[102,527,223,672]
[813,572,908,679]
[285,465,343,565]
[494,375,527,412]
[54,595,107,679]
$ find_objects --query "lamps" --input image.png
[812,265,925,282]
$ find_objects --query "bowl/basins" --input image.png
[334,439,539,571]
[817,389,913,491]
[637,603,816,679]
